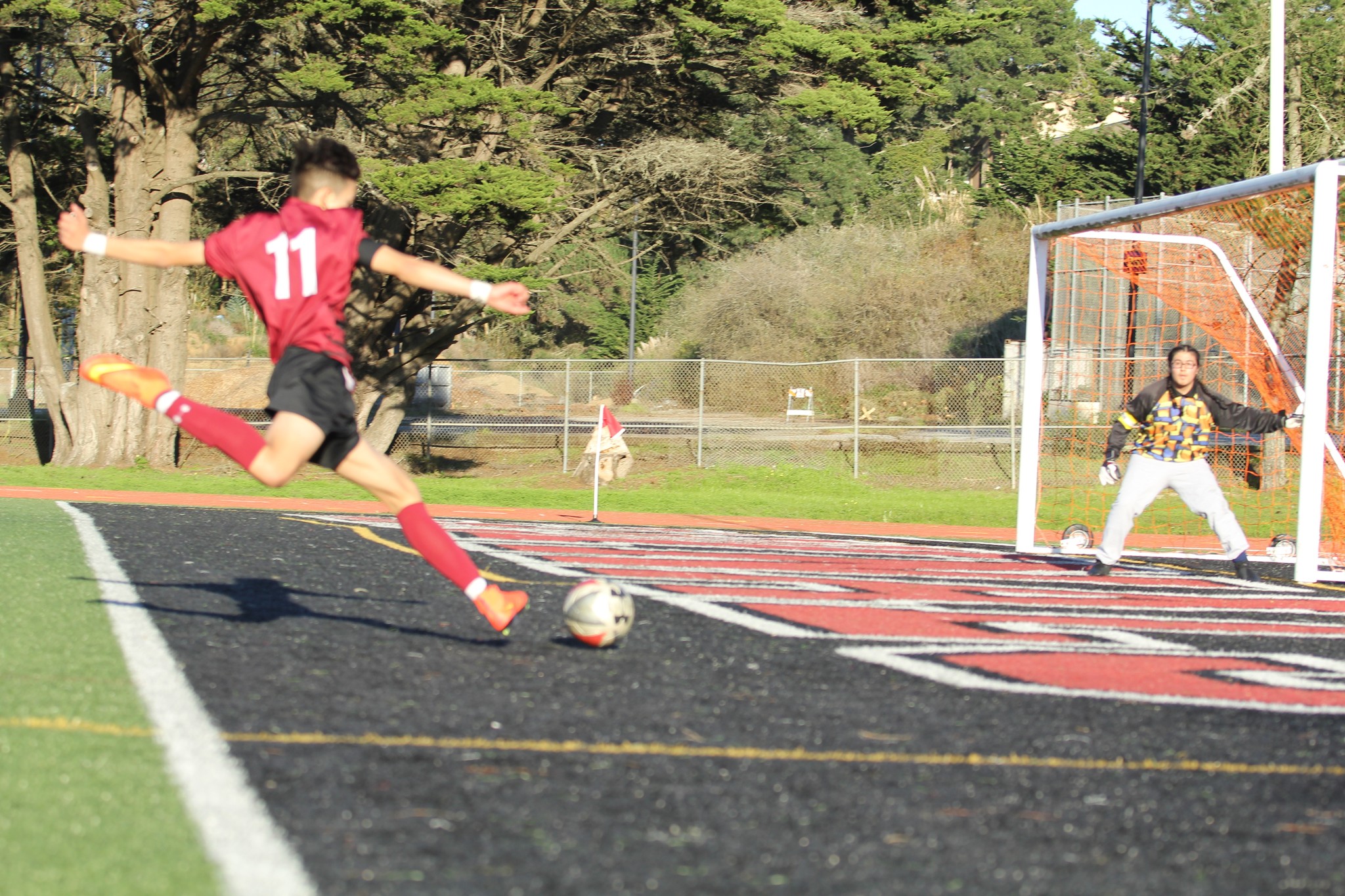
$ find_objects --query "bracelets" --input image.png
[83,232,107,257]
[470,280,491,305]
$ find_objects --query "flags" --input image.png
[602,407,626,441]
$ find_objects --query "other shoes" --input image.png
[1088,559,1111,577]
[1234,560,1251,580]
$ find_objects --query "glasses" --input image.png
[1169,361,1198,369]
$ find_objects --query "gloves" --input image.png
[1099,460,1122,485]
[1285,403,1306,428]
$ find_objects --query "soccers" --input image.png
[562,577,636,648]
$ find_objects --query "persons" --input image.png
[58,138,537,637]
[1087,345,1303,582]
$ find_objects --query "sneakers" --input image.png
[473,585,530,635]
[80,353,174,408]
